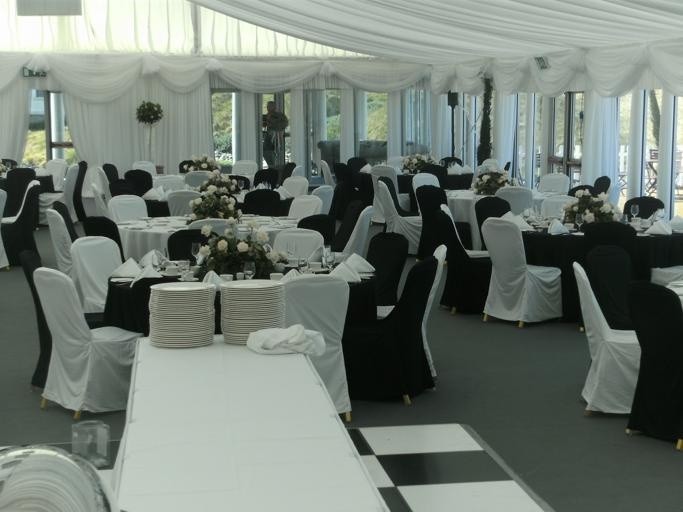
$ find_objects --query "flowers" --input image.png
[138,100,164,124]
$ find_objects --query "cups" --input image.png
[632,217,641,224]
[165,243,334,281]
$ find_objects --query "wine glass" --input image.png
[574,214,583,233]
[631,205,639,225]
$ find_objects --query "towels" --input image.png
[245,324,328,358]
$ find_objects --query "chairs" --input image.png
[3,159,450,425]
[316,155,678,428]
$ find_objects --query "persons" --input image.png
[262,101,288,169]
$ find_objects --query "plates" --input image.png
[117,213,297,231]
[110,277,133,283]
[160,272,181,277]
[220,279,286,346]
[147,281,216,349]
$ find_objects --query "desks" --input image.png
[111,334,392,512]
